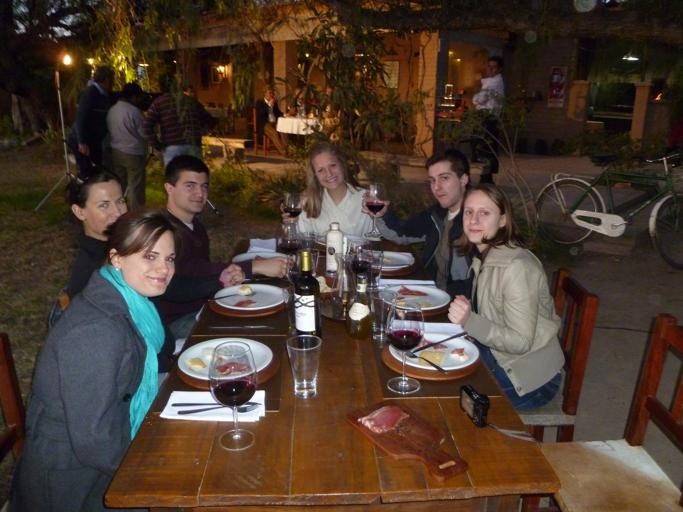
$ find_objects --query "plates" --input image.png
[387,333,480,370]
[358,251,414,271]
[215,284,287,311]
[177,337,273,381]
[378,285,451,311]
[231,251,287,263]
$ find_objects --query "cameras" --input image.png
[460,384,490,427]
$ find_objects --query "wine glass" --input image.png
[284,192,302,230]
[364,184,385,237]
[385,302,423,395]
[208,342,257,451]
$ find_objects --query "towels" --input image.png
[248,239,281,252]
[357,248,415,266]
[391,318,466,342]
[376,277,436,290]
[159,389,265,423]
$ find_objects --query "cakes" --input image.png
[419,351,445,366]
[187,357,206,371]
[237,286,252,295]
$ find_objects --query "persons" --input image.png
[254,55,378,150]
[281,142,385,246]
[162,154,295,337]
[102,80,147,211]
[64,168,245,389]
[143,73,216,174]
[471,55,508,173]
[9,210,178,512]
[447,184,565,411]
[67,65,123,182]
[360,149,477,311]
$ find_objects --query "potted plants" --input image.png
[230,46,253,135]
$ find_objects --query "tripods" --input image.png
[33,77,87,213]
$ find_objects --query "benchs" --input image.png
[202,137,252,163]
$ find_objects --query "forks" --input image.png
[177,404,257,419]
[404,351,447,375]
[207,292,255,303]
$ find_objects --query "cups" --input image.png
[345,240,368,273]
[368,290,398,349]
[278,223,300,253]
[284,335,322,399]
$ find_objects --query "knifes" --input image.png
[172,402,261,407]
[411,332,468,354]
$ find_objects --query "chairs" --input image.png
[58,291,69,310]
[516,268,600,442]
[521,312,683,512]
[253,107,269,155]
[0,332,26,512]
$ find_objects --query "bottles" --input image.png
[291,251,323,350]
[324,223,345,277]
[331,254,357,321]
[346,273,369,340]
[297,232,318,278]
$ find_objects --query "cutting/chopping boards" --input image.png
[344,399,468,482]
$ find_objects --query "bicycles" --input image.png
[534,152,683,265]
